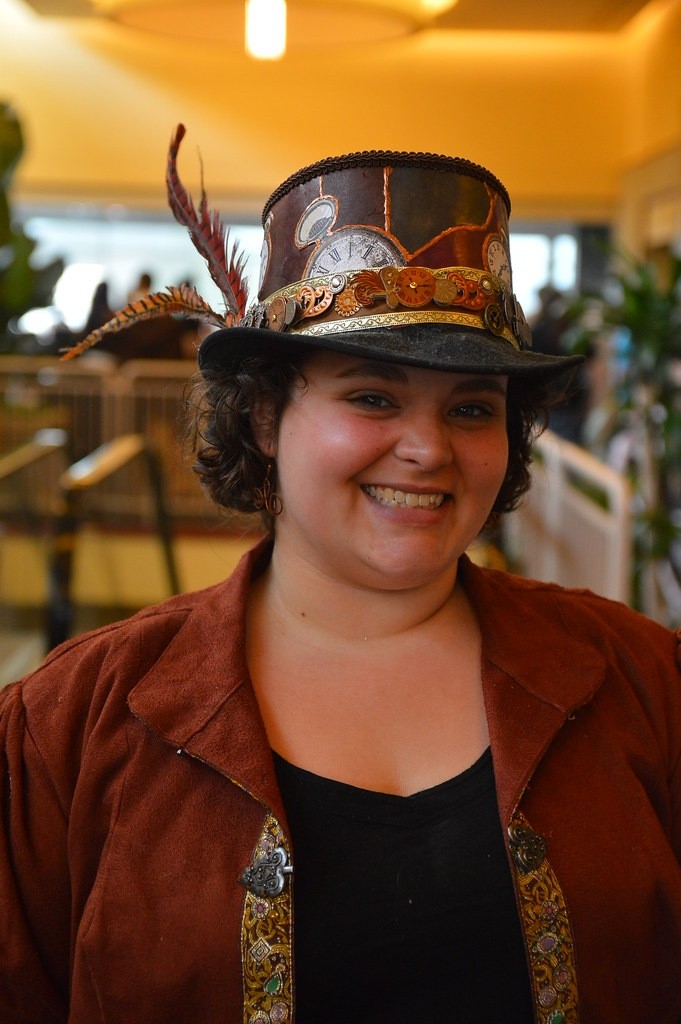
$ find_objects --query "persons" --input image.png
[0,123,681,1024]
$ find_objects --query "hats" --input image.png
[57,120,589,379]
